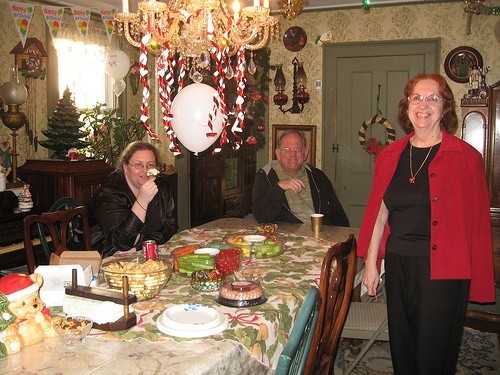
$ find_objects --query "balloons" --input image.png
[104,50,129,82]
[169,83,224,156]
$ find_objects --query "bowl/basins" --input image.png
[224,232,286,258]
[171,243,244,279]
[100,257,174,302]
[53,314,95,343]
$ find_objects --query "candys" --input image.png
[192,269,223,290]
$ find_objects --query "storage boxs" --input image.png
[36,249,102,305]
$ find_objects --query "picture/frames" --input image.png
[271,124,317,166]
[444,46,484,84]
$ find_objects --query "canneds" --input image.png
[143,239,158,261]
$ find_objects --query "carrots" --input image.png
[172,246,197,270]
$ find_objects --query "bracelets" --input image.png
[136,200,147,211]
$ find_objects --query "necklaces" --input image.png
[409,137,434,184]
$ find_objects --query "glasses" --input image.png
[128,161,155,170]
[278,148,303,153]
[407,93,446,105]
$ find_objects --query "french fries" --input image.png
[103,259,169,298]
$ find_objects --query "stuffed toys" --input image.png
[0,273,62,354]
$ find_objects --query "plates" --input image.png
[283,27,308,51]
[156,303,227,338]
[217,281,267,307]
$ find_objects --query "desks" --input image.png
[0,217,364,375]
[17,158,113,204]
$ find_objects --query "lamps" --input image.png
[0,63,28,187]
[274,57,310,113]
[106,0,311,157]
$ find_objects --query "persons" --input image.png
[469,65,481,98]
[357,73,496,375]
[250,129,350,227]
[0,135,15,178]
[68,140,177,259]
[0,156,8,191]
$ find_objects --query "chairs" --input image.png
[339,273,390,375]
[23,195,89,272]
[274,234,355,375]
[464,213,500,324]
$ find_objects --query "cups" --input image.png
[311,213,323,231]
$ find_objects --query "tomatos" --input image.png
[216,249,241,276]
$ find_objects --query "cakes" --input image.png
[220,281,262,300]
